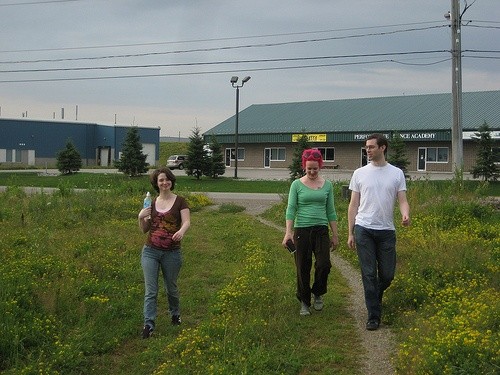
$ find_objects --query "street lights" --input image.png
[230,76,251,179]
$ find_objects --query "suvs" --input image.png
[167,155,188,170]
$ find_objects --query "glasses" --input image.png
[304,153,320,158]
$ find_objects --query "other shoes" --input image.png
[143,326,153,339]
[366,319,379,330]
[300,302,311,316]
[313,296,324,311]
[171,315,182,325]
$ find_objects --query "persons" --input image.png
[282,149,338,316]
[138,169,191,338]
[347,134,410,329]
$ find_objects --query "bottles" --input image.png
[144,192,151,220]
